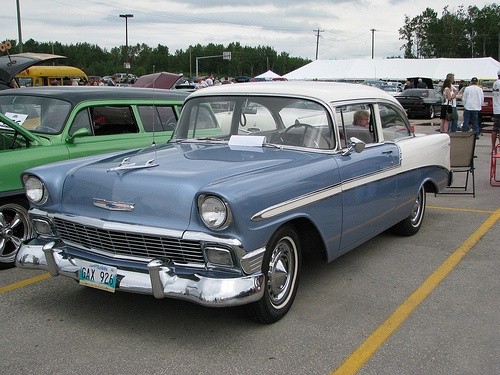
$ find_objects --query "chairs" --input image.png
[433,131,478,197]
[487,131,500,187]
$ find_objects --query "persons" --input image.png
[8,79,33,118]
[462,77,484,140]
[432,79,465,93]
[249,78,257,82]
[379,78,392,85]
[353,110,370,128]
[84,75,118,86]
[404,79,415,90]
[50,80,61,86]
[194,74,235,89]
[492,71,500,154]
[438,74,459,133]
[416,78,428,89]
[398,82,406,92]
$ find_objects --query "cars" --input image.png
[0,85,224,270]
[15,80,451,327]
[480,89,494,117]
[362,80,447,120]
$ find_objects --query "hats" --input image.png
[497,69,500,76]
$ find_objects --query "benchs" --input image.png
[94,123,138,135]
[268,126,405,145]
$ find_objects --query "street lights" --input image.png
[118,13,135,74]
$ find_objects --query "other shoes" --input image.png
[490,147,497,155]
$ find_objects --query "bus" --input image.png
[16,66,89,87]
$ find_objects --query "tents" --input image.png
[252,70,282,81]
[281,57,500,81]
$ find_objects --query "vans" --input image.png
[116,73,134,82]
